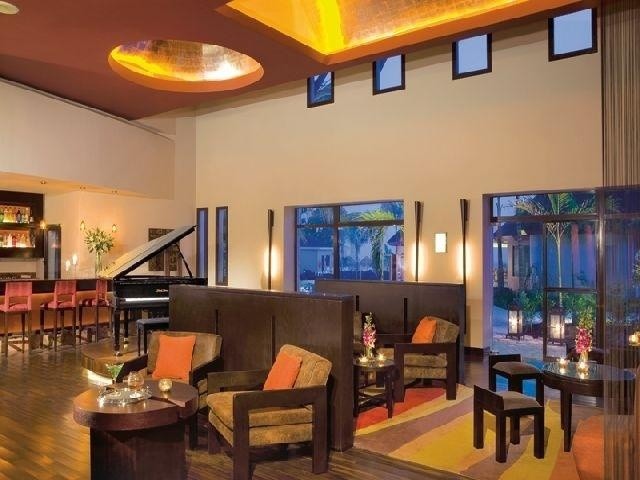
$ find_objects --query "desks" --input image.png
[545,359,637,453]
[74,378,199,476]
[351,352,394,420]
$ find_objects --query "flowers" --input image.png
[574,308,596,360]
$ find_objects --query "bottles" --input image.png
[0,206,35,224]
[0,232,34,249]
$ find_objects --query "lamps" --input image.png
[628,333,640,352]
[550,308,567,349]
[505,308,525,341]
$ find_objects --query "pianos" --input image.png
[96,223,208,349]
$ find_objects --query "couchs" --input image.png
[114,330,226,451]
[205,344,334,475]
[372,314,461,405]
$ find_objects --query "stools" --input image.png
[487,352,544,402]
[472,383,546,464]
[137,316,178,362]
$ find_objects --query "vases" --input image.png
[576,354,593,380]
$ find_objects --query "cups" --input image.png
[159,378,173,392]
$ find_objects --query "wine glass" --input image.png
[128,371,144,399]
[104,362,124,397]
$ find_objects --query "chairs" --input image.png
[2,280,34,357]
[80,274,113,346]
[39,275,78,352]
